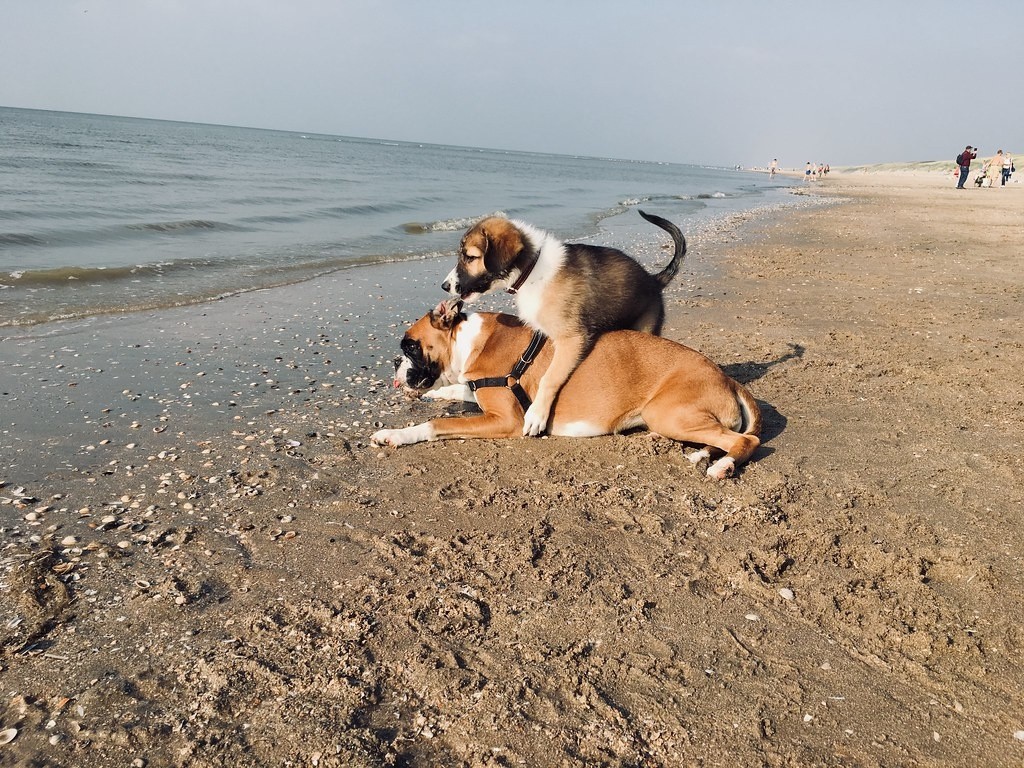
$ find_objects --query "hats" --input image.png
[966,146,973,149]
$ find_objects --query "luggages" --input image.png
[981,170,992,188]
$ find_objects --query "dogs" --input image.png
[370,301,763,478]
[441,210,686,436]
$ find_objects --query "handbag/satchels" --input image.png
[1010,159,1016,172]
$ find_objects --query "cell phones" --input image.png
[974,148,977,151]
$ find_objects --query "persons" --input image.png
[770,158,779,177]
[819,164,829,177]
[803,162,816,181]
[957,146,976,189]
[980,150,1012,187]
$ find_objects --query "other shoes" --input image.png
[956,186,966,189]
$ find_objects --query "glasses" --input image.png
[1001,153,1003,154]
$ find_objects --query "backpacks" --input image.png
[956,152,968,165]
